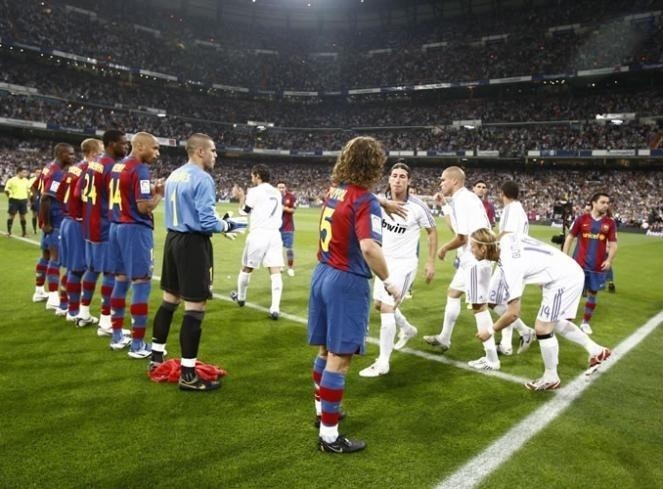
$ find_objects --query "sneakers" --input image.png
[34,286,176,370]
[8,232,38,239]
[229,290,246,308]
[177,375,221,392]
[314,410,366,453]
[360,283,615,391]
[289,268,296,279]
[266,311,280,318]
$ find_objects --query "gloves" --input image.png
[221,211,248,243]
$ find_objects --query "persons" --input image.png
[421,166,612,392]
[0,0,662,236]
[359,163,438,377]
[230,166,283,320]
[147,134,249,392]
[5,168,41,236]
[278,181,296,276]
[33,130,164,359]
[563,192,618,334]
[307,136,409,454]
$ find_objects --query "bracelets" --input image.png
[383,277,394,288]
